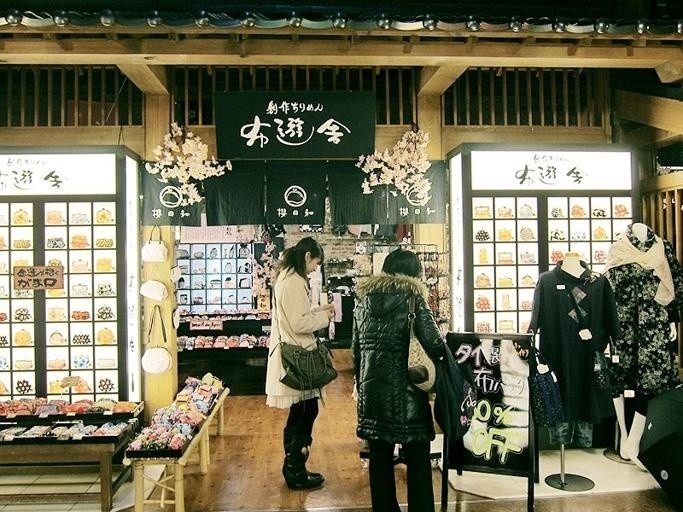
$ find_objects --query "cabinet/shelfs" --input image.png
[173,220,271,395]
[446,141,643,452]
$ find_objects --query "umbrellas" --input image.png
[637,384,682,512]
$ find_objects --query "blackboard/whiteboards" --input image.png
[443,332,536,477]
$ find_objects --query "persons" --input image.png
[349,249,447,512]
[526,253,620,447]
[604,223,682,471]
[263,237,336,490]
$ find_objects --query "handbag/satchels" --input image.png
[280,342,336,391]
[141,240,168,263]
[408,330,436,392]
[589,351,621,397]
[527,371,562,430]
[141,347,173,374]
[140,280,168,302]
[434,338,474,440]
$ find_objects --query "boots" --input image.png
[282,427,325,489]
